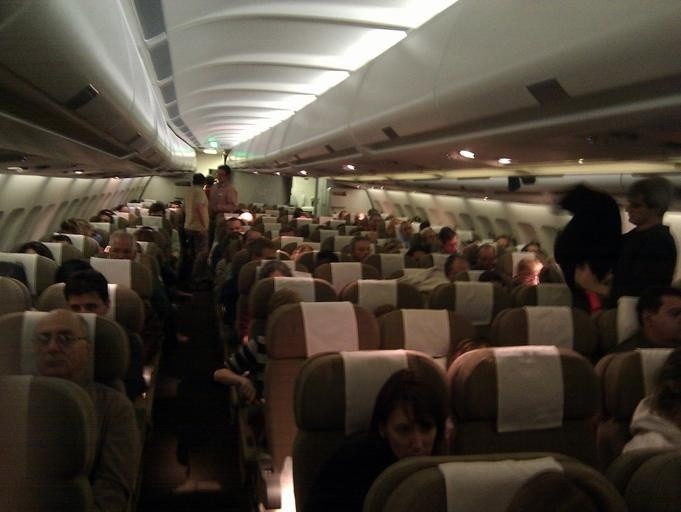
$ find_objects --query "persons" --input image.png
[350,370,468,491]
[615,286,680,354]
[446,332,495,370]
[215,232,567,323]
[208,165,237,231]
[507,469,609,512]
[204,176,215,199]
[0,200,185,511]
[620,347,681,455]
[209,288,302,439]
[185,174,210,273]
[224,217,244,232]
[577,176,677,306]
[239,200,431,233]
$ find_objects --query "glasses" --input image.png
[354,247,371,252]
[215,172,227,178]
[478,255,496,264]
[31,332,88,347]
